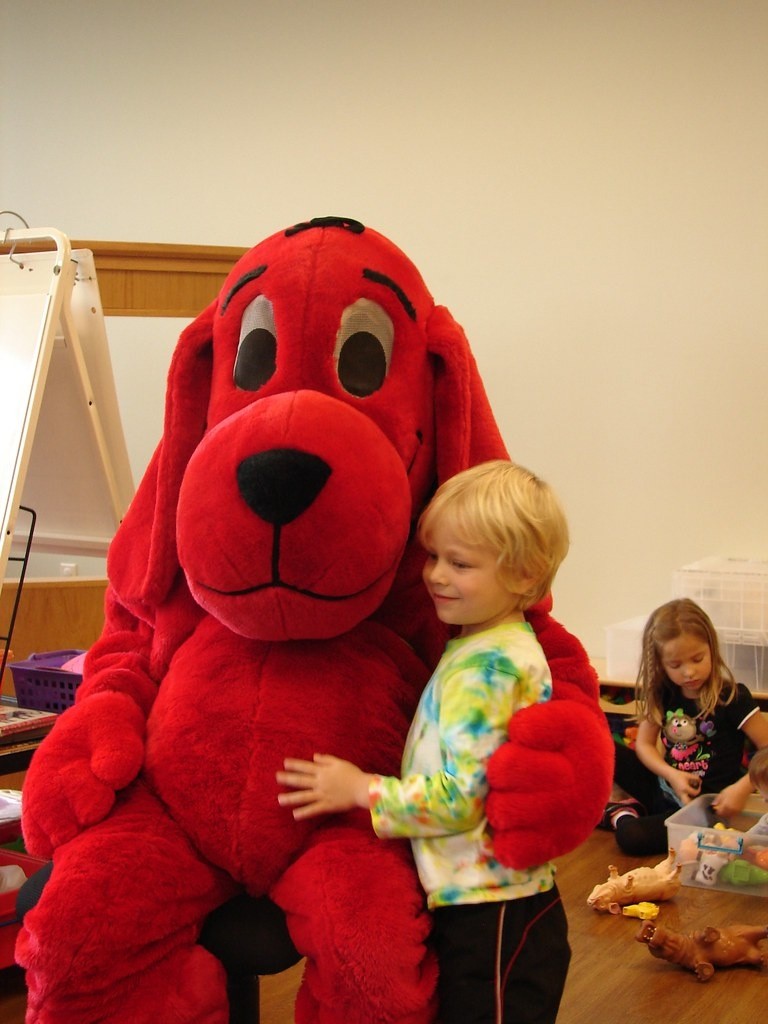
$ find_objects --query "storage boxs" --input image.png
[608,551,768,694]
[664,794,768,898]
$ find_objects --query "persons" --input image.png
[277,460,570,1024]
[744,747,768,843]
[594,597,768,855]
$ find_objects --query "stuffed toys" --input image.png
[14,214,614,1022]
[663,713,707,781]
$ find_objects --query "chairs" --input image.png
[15,858,306,1024]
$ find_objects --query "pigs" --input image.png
[586,847,682,915]
